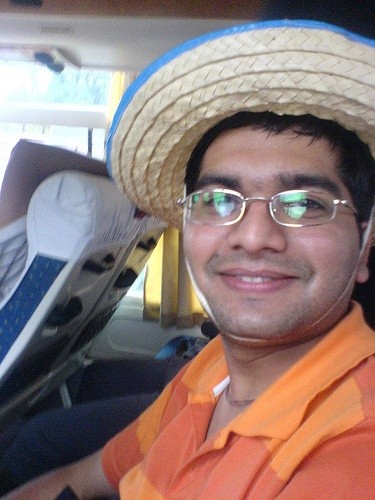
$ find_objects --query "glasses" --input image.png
[177,189,362,227]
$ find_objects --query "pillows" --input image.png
[0,138,112,224]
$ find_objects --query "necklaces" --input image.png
[225,384,255,406]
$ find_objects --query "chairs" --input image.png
[0,172,169,422]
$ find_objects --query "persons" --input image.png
[0,138,151,302]
[0,20,375,500]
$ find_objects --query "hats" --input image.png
[106,19,375,228]
